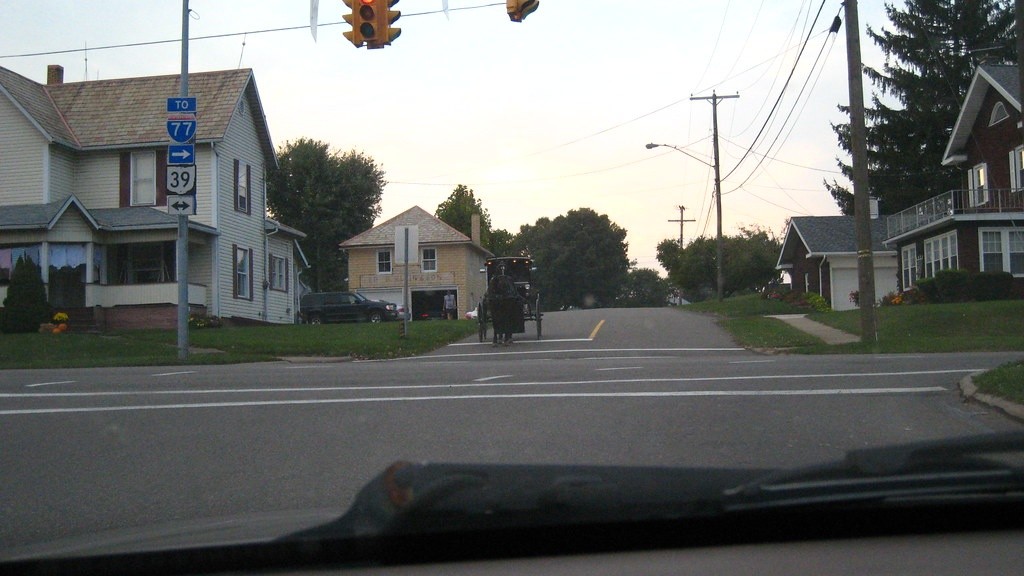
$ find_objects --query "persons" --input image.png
[442,290,457,320]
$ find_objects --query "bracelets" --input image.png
[454,305,456,306]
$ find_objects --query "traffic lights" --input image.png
[342,0,354,44]
[353,0,387,44]
[506,2,540,23]
[388,0,402,45]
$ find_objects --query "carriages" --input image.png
[476,256,544,347]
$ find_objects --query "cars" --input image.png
[371,298,412,321]
[466,305,491,322]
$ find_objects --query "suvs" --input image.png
[299,291,398,326]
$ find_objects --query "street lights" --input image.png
[645,142,725,302]
